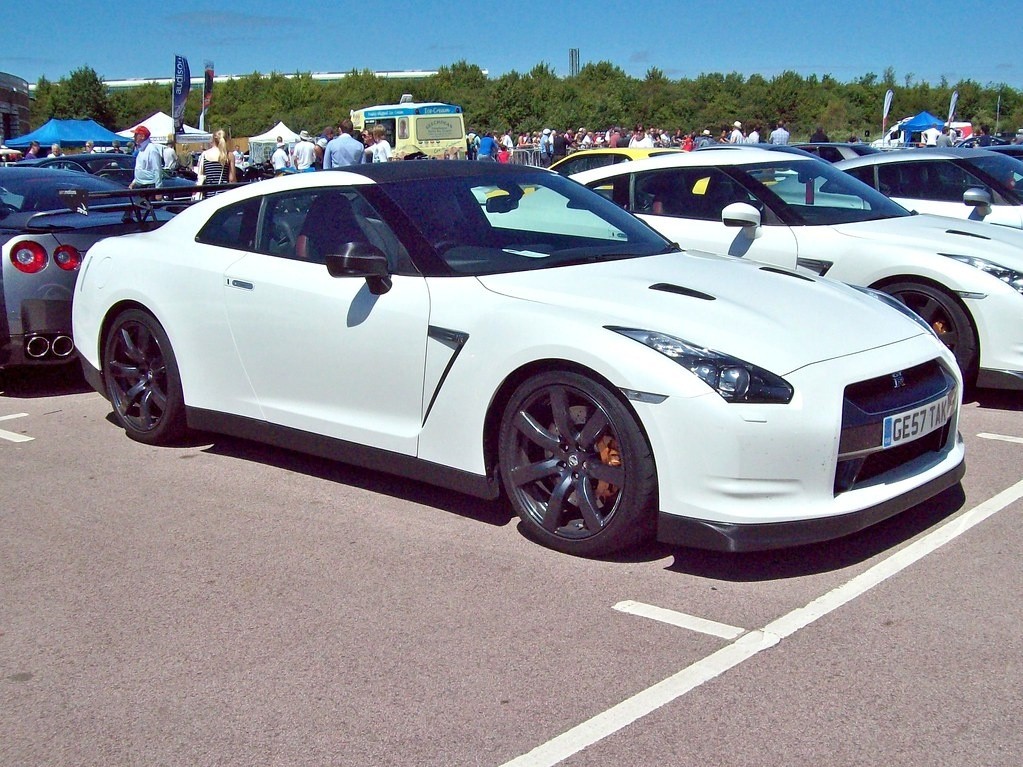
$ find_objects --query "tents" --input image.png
[248,122,317,168]
[102,111,213,151]
[897,111,944,147]
[5,119,136,153]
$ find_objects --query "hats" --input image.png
[299,130,313,140]
[703,130,710,135]
[276,143,285,148]
[543,129,551,135]
[130,126,150,138]
[731,120,742,129]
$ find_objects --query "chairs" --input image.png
[649,172,690,218]
[295,195,370,271]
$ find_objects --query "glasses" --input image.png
[362,134,370,138]
[636,130,643,132]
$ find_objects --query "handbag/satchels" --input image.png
[191,175,207,201]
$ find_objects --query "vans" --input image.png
[350,94,469,161]
[868,116,972,150]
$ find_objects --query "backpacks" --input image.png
[466,135,478,152]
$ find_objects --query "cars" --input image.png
[0,165,254,383]
[72,158,966,559]
[10,152,202,214]
[482,142,1023,404]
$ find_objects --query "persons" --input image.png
[399,120,407,137]
[810,127,829,143]
[1013,129,1023,146]
[671,121,762,152]
[198,130,239,199]
[921,123,961,148]
[85,140,96,153]
[270,119,393,178]
[467,128,514,163]
[0,141,71,172]
[516,124,670,168]
[847,132,858,144]
[232,145,245,168]
[106,126,179,201]
[767,121,791,145]
[974,124,1001,147]
